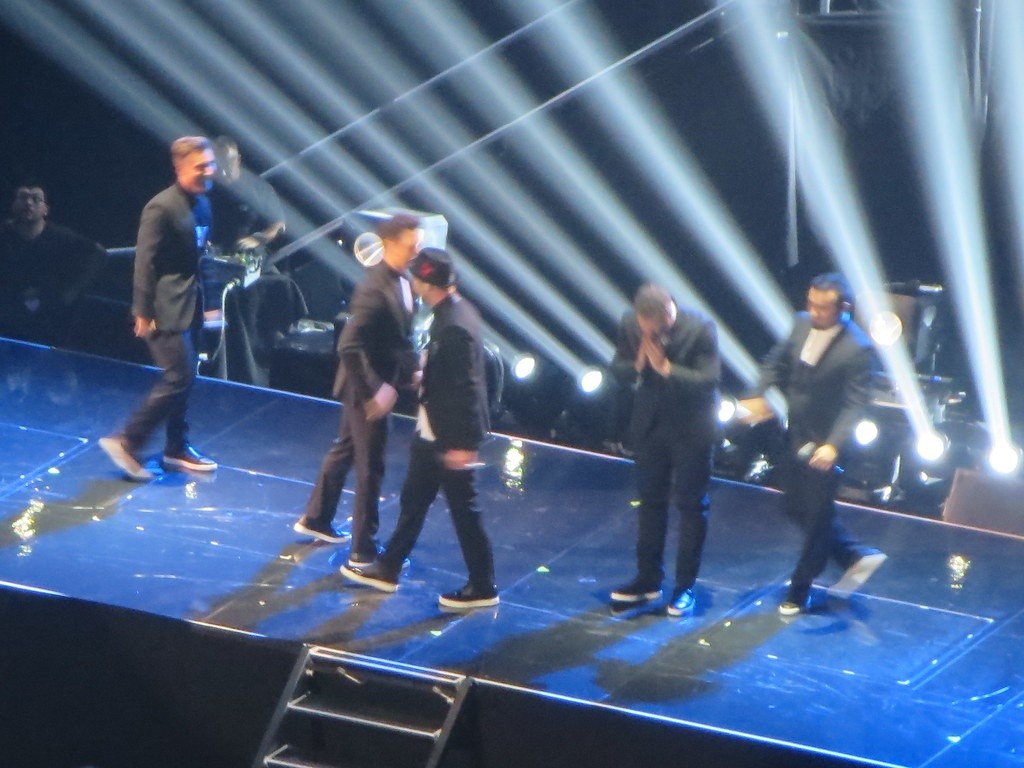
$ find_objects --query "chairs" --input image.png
[198,273,332,399]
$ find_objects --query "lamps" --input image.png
[912,430,953,480]
[577,364,620,406]
[509,352,550,405]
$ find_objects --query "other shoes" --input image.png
[668,585,696,616]
[779,596,812,615]
[611,578,663,602]
[340,559,399,593]
[439,580,500,608]
[348,546,410,569]
[294,514,352,544]
[163,443,218,472]
[99,435,153,479]
[829,553,887,598]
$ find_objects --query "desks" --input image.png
[271,329,340,369]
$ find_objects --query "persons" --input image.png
[99,135,218,478]
[610,284,721,616]
[293,214,499,608]
[761,272,888,615]
[0,135,285,351]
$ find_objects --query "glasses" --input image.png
[385,239,423,252]
[15,194,48,204]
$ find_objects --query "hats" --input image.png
[408,247,457,287]
